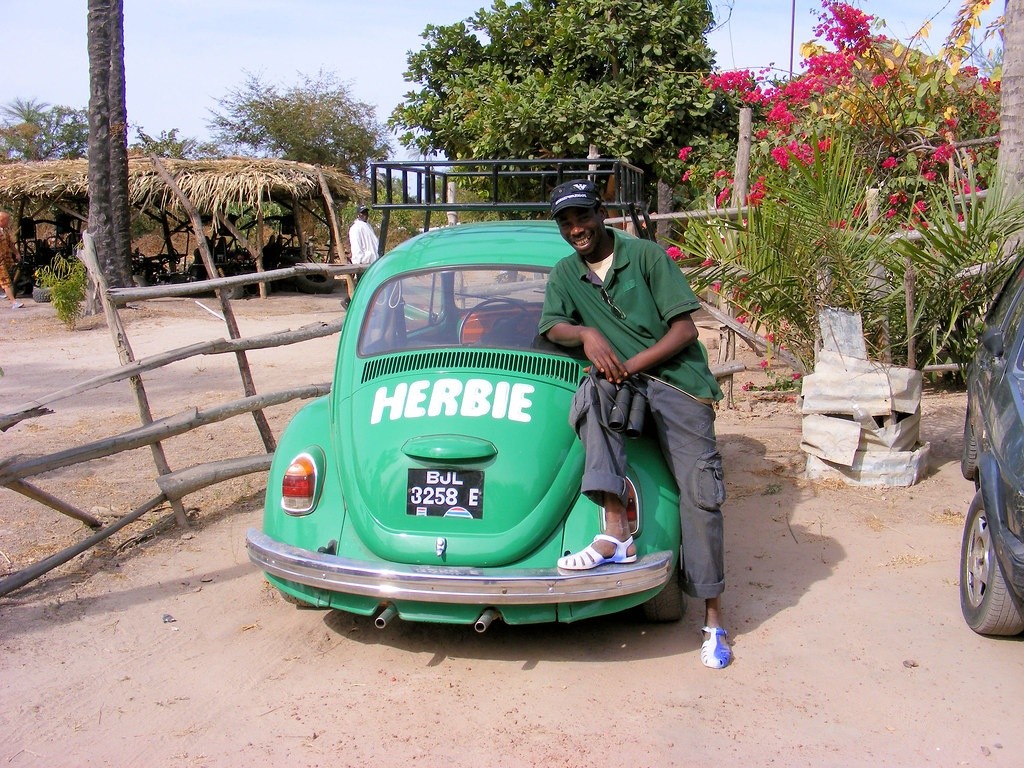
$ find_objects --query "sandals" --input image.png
[700,627,731,668]
[557,534,637,569]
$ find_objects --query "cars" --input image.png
[958,250,1024,641]
[244,218,687,633]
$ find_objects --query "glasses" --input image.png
[599,286,627,320]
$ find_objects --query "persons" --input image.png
[0,212,24,308]
[538,179,732,668]
[341,206,380,310]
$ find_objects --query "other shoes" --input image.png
[341,299,349,310]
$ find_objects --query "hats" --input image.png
[358,204,369,213]
[548,179,605,220]
